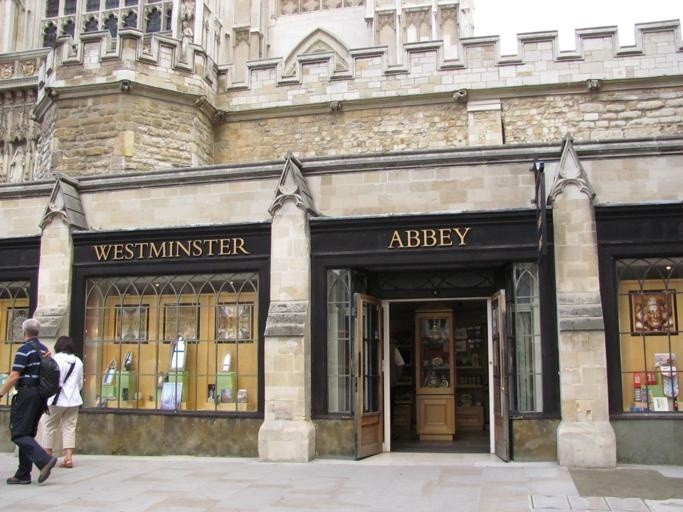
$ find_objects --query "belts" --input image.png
[20,384,36,388]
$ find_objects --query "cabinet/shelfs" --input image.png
[215,371,237,403]
[390,319,413,432]
[101,371,135,401]
[455,311,487,432]
[167,371,188,401]
[414,302,456,441]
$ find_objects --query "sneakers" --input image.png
[7,475,31,484]
[39,456,57,482]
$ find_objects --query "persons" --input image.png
[0,319,60,484]
[42,336,83,468]
[636,297,674,332]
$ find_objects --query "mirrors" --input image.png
[101,358,116,385]
[168,331,187,371]
[122,351,133,371]
[220,351,231,372]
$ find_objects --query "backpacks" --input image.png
[26,341,60,399]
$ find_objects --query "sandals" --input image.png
[59,459,73,468]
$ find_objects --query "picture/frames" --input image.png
[162,303,199,344]
[213,301,253,343]
[113,303,148,344]
[4,306,30,344]
[628,289,678,336]
